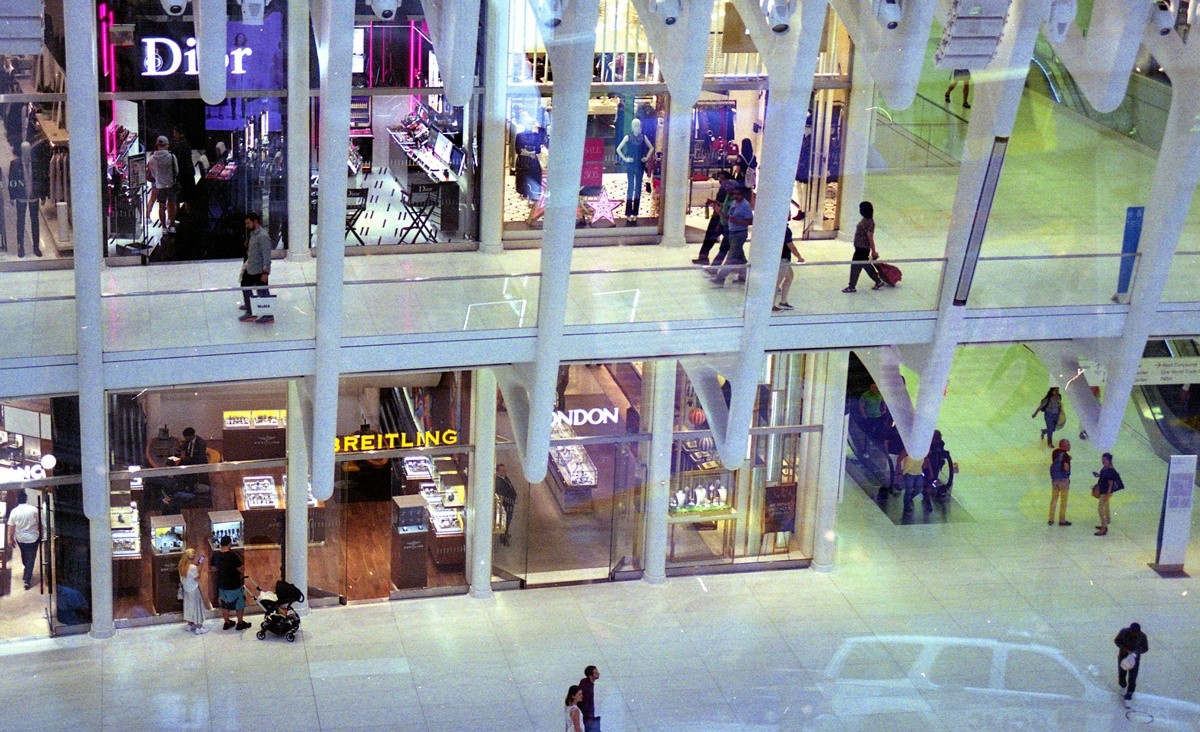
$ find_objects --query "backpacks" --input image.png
[1040,394,1061,411]
[740,152,756,188]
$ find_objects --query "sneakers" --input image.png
[224,620,236,630]
[186,624,209,634]
[235,621,251,630]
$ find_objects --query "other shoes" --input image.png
[1048,520,1054,525]
[872,282,885,291]
[842,287,857,292]
[692,258,795,311]
[238,314,258,322]
[1041,429,1045,440]
[239,305,247,310]
[1095,526,1108,531]
[1121,686,1127,695]
[256,315,275,324]
[1125,699,1131,709]
[1094,531,1106,535]
[24,582,30,589]
[1058,520,1071,526]
[1048,443,1055,448]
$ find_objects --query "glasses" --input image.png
[730,206,735,214]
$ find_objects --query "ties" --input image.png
[188,442,193,458]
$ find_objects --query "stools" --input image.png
[340,188,369,246]
[400,190,439,244]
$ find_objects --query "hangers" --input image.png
[52,144,69,158]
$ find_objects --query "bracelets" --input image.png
[263,272,268,274]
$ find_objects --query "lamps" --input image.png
[160,0,191,16]
[759,0,797,37]
[365,0,402,21]
[534,0,569,27]
[237,0,272,26]
[648,0,684,26]
[873,0,904,29]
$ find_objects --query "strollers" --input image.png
[242,575,305,642]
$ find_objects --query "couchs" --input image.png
[687,177,719,207]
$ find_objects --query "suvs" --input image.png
[798,633,1199,730]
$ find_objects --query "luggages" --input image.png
[873,256,903,287]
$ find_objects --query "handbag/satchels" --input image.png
[250,281,278,315]
[1111,473,1124,493]
[1121,653,1136,670]
[1050,451,1071,479]
[1092,484,1100,498]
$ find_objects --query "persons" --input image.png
[736,138,756,202]
[945,69,971,108]
[793,119,812,220]
[859,375,944,514]
[557,365,569,412]
[617,119,654,222]
[692,170,804,313]
[516,117,542,201]
[161,427,206,516]
[178,537,251,634]
[1113,623,1148,708]
[496,464,517,546]
[146,123,196,233]
[7,141,46,258]
[1031,386,1112,536]
[239,212,275,324]
[565,665,600,732]
[842,201,885,293]
[8,493,43,587]
